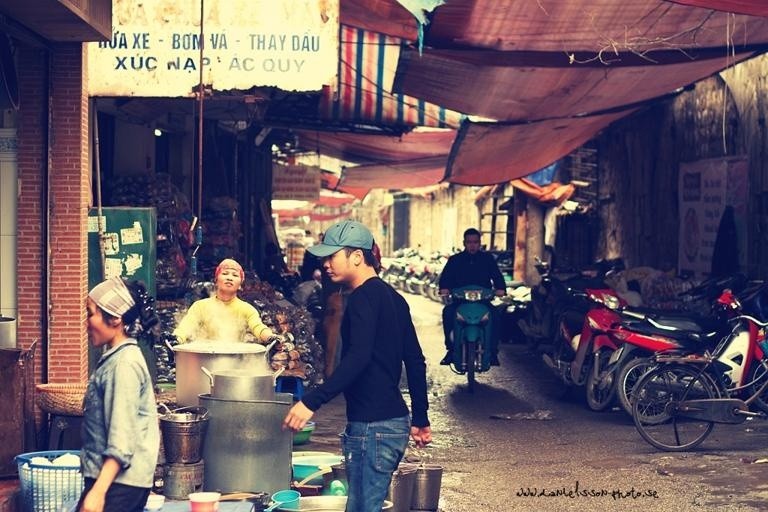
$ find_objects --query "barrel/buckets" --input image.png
[160,405,211,463]
[392,447,442,512]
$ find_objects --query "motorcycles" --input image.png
[608,273,768,424]
[381,243,462,303]
[518,252,626,412]
[442,286,514,389]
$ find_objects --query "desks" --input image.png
[47,415,89,451]
[145,502,255,512]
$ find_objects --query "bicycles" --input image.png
[632,315,768,451]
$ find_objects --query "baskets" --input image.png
[15,450,86,512]
[36,382,88,417]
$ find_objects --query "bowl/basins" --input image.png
[293,421,316,445]
[291,450,345,485]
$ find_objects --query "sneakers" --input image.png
[441,350,452,365]
[490,356,500,366]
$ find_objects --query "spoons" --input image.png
[264,490,301,512]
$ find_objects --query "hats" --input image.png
[306,220,375,257]
[216,258,246,284]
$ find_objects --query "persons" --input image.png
[175,258,278,346]
[74,276,162,512]
[285,230,381,295]
[439,228,507,365]
[281,221,432,512]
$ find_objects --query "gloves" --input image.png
[269,334,284,351]
[166,334,180,345]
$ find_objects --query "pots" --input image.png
[201,366,285,402]
[165,338,277,410]
